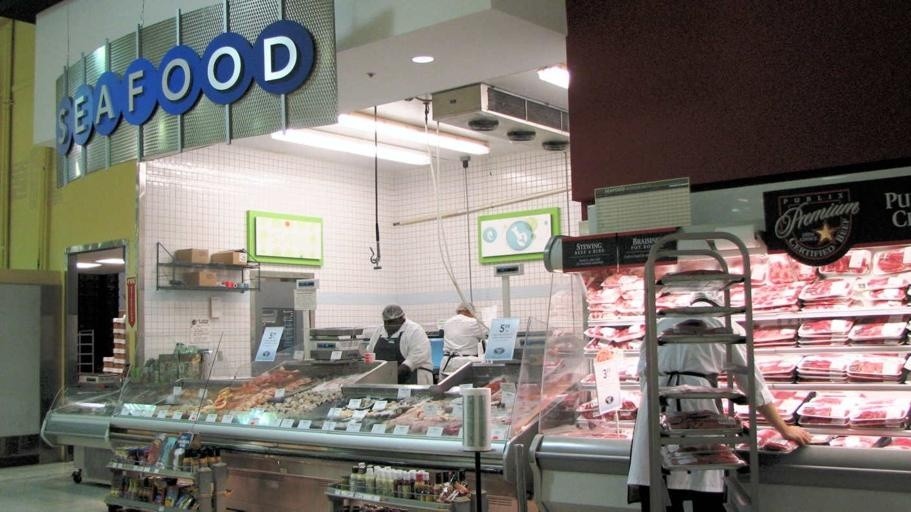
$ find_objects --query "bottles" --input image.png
[107,441,221,508]
[139,342,201,383]
[340,462,469,512]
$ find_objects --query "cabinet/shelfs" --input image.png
[575,241,911,449]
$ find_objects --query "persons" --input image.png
[437,301,488,383]
[623,291,811,512]
[364,304,433,386]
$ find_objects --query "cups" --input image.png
[364,353,375,364]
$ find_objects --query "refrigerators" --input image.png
[525,229,911,512]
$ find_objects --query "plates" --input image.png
[575,249,911,451]
